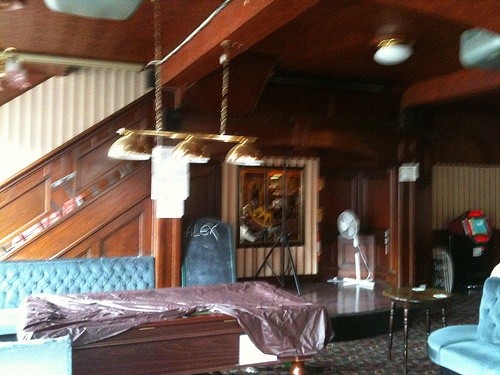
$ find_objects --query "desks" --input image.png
[18,280,326,375]
[382,287,452,375]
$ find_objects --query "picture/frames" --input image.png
[237,166,305,248]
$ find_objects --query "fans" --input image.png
[338,212,375,289]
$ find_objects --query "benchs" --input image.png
[0,338,73,375]
[0,256,155,336]
[428,277,500,375]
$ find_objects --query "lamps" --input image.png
[106,1,262,165]
[150,145,190,219]
[369,34,413,65]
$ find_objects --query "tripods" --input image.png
[250,162,302,297]
